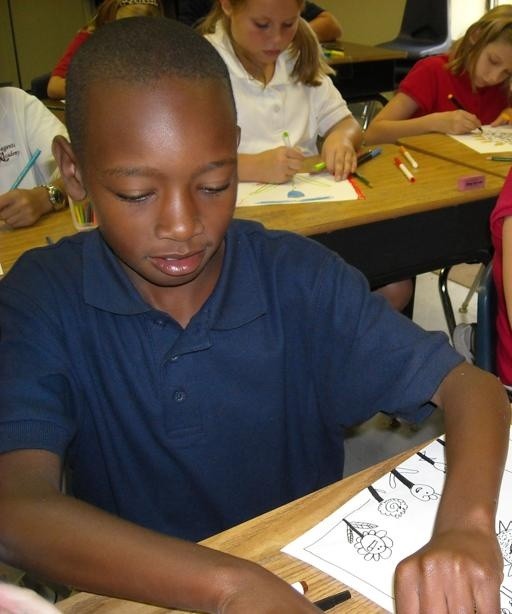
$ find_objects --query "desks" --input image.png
[0,1,512,431]
[51,401,512,614]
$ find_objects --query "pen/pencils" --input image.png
[448,94,484,132]
[73,198,98,227]
[400,146,418,168]
[486,156,512,162]
[331,147,382,200]
[291,580,309,593]
[314,161,326,170]
[9,148,41,193]
[320,44,347,58]
[394,157,416,182]
[281,131,292,148]
[312,589,352,613]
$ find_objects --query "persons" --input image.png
[0,17,512,614]
[300,0,342,43]
[47,0,164,101]
[190,0,412,315]
[365,5,512,145]
[0,86,71,228]
[488,165,512,402]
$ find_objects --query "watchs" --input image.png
[33,184,66,212]
